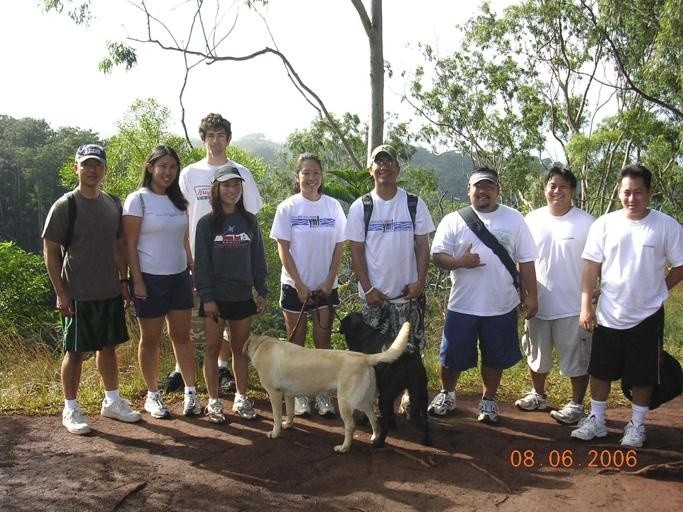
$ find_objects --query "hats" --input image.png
[371,145,397,162]
[209,167,246,183]
[75,144,106,166]
[469,171,499,188]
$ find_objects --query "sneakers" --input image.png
[478,400,498,423]
[63,395,258,434]
[428,389,456,416]
[315,393,336,416]
[218,367,236,391]
[571,413,608,440]
[162,371,183,394]
[399,396,411,416]
[621,419,647,447]
[515,388,547,411]
[549,401,584,424]
[294,397,311,415]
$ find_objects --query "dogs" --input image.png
[239,320,411,455]
[338,310,430,448]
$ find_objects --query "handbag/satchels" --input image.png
[620,350,682,411]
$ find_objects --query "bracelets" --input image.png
[119,278,129,284]
[364,285,374,295]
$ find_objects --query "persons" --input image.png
[188,164,273,422]
[39,141,141,435]
[266,148,349,418]
[425,164,539,423]
[118,144,203,420]
[339,143,433,419]
[155,111,265,392]
[568,159,683,449]
[512,166,600,425]
